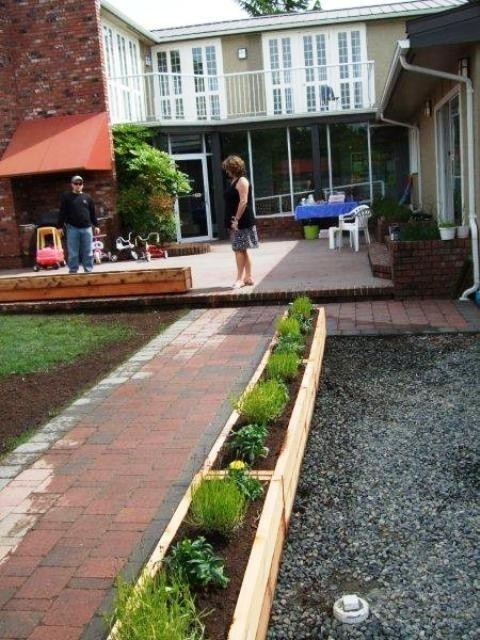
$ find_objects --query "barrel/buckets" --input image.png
[303,225,319,240]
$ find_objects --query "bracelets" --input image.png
[231,216,239,220]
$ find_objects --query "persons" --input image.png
[222,155,259,289]
[55,176,100,274]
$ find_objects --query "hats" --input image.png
[71,175,83,182]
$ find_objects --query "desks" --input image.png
[295,200,359,239]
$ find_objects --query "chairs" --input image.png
[337,203,374,252]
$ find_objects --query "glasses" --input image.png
[73,182,83,185]
